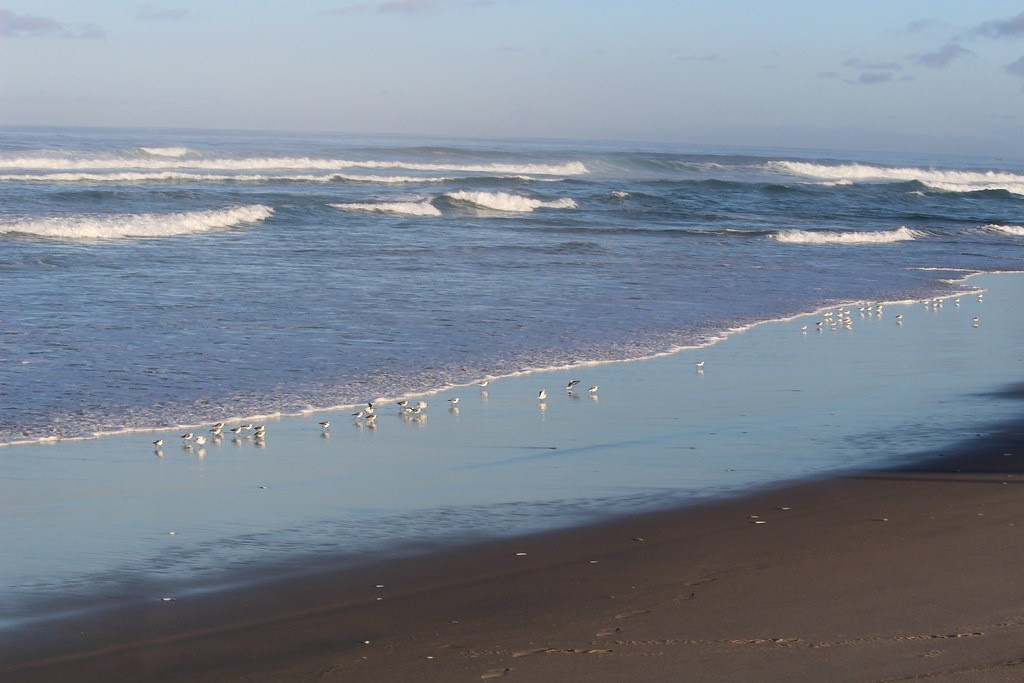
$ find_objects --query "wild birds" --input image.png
[536,380,599,402]
[152,422,266,447]
[694,360,706,368]
[919,293,983,308]
[351,401,379,420]
[972,317,979,323]
[397,400,429,416]
[448,396,461,404]
[800,305,904,331]
[476,379,488,387]
[319,420,332,429]
